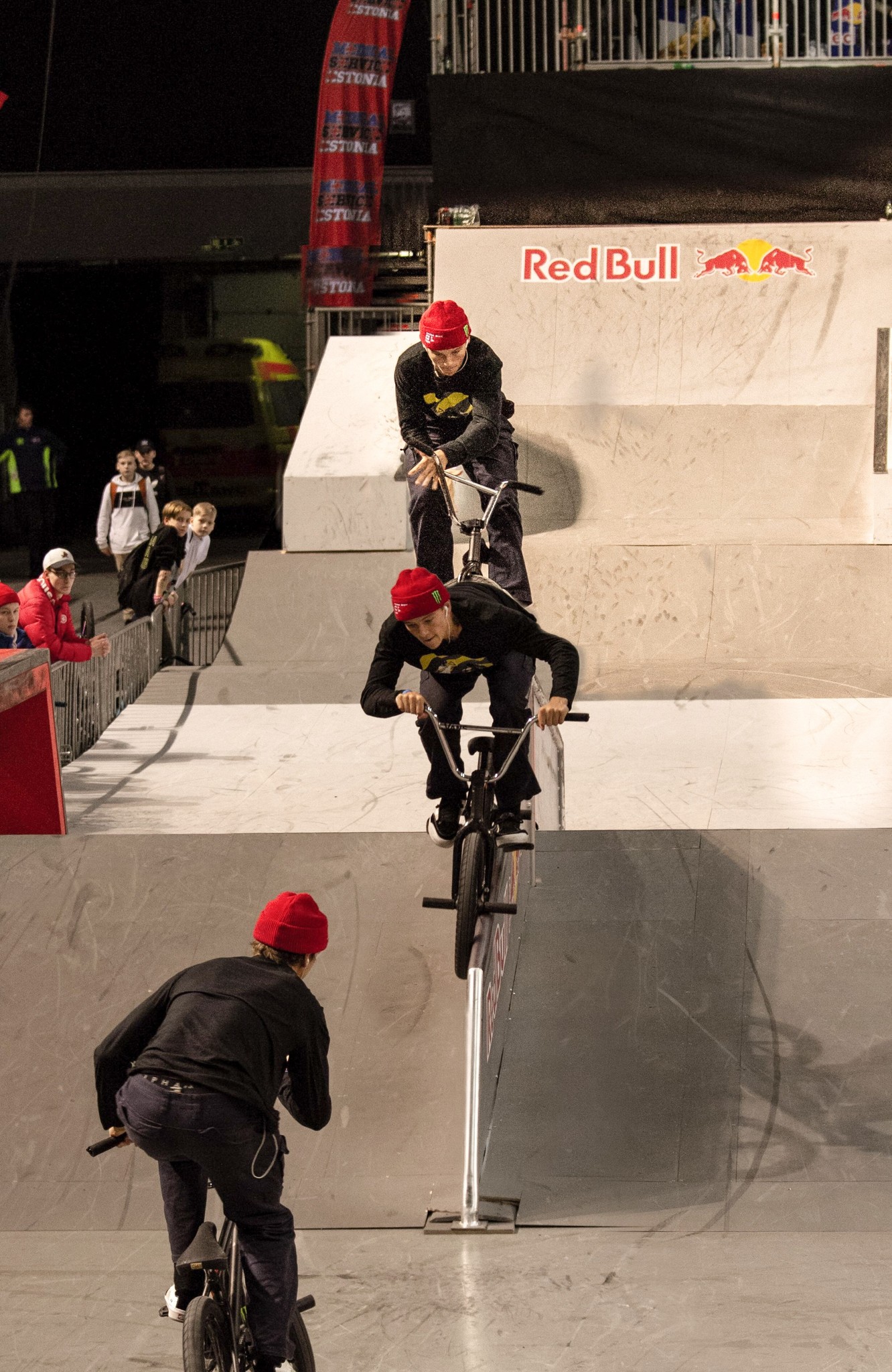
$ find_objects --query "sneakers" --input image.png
[492,809,531,847]
[164,1282,188,1322]
[428,794,464,847]
[253,1353,295,1372]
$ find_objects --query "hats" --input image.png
[391,568,451,620]
[136,439,153,453]
[43,548,81,573]
[254,891,329,955]
[0,582,21,608]
[419,300,472,351]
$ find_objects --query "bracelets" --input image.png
[154,595,162,602]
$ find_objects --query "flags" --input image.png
[308,0,408,250]
[301,245,373,308]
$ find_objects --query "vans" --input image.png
[155,340,305,538]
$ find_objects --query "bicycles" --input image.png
[86,1131,315,1372]
[408,438,544,590]
[377,696,590,979]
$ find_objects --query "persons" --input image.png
[0,405,65,580]
[359,568,579,848]
[18,548,112,663]
[94,451,160,621]
[0,583,36,649]
[134,441,166,508]
[119,501,193,666]
[171,502,217,601]
[92,892,331,1372]
[394,300,533,608]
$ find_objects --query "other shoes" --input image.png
[124,614,134,624]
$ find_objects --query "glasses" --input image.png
[48,569,79,580]
